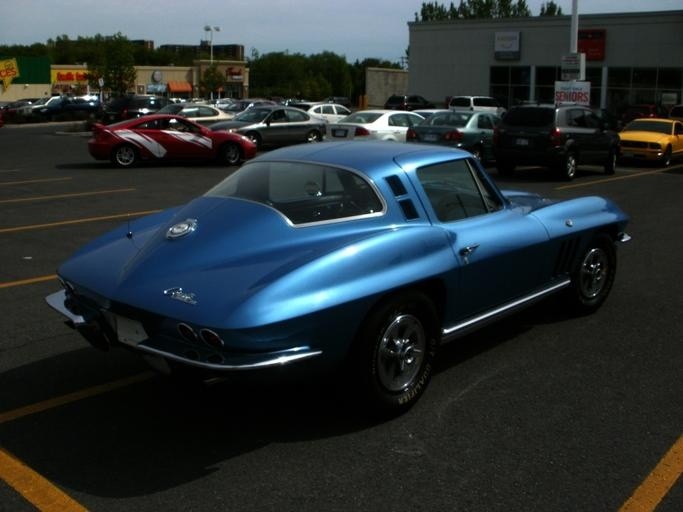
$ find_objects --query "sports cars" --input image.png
[41,133,634,417]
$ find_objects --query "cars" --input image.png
[208,106,327,150]
[1,89,292,128]
[411,108,449,118]
[323,110,426,142]
[615,118,683,167]
[292,101,354,122]
[85,114,257,170]
[405,109,499,155]
[146,103,236,130]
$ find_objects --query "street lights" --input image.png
[203,24,221,66]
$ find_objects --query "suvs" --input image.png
[492,104,622,180]
[447,96,506,116]
[384,95,437,110]
